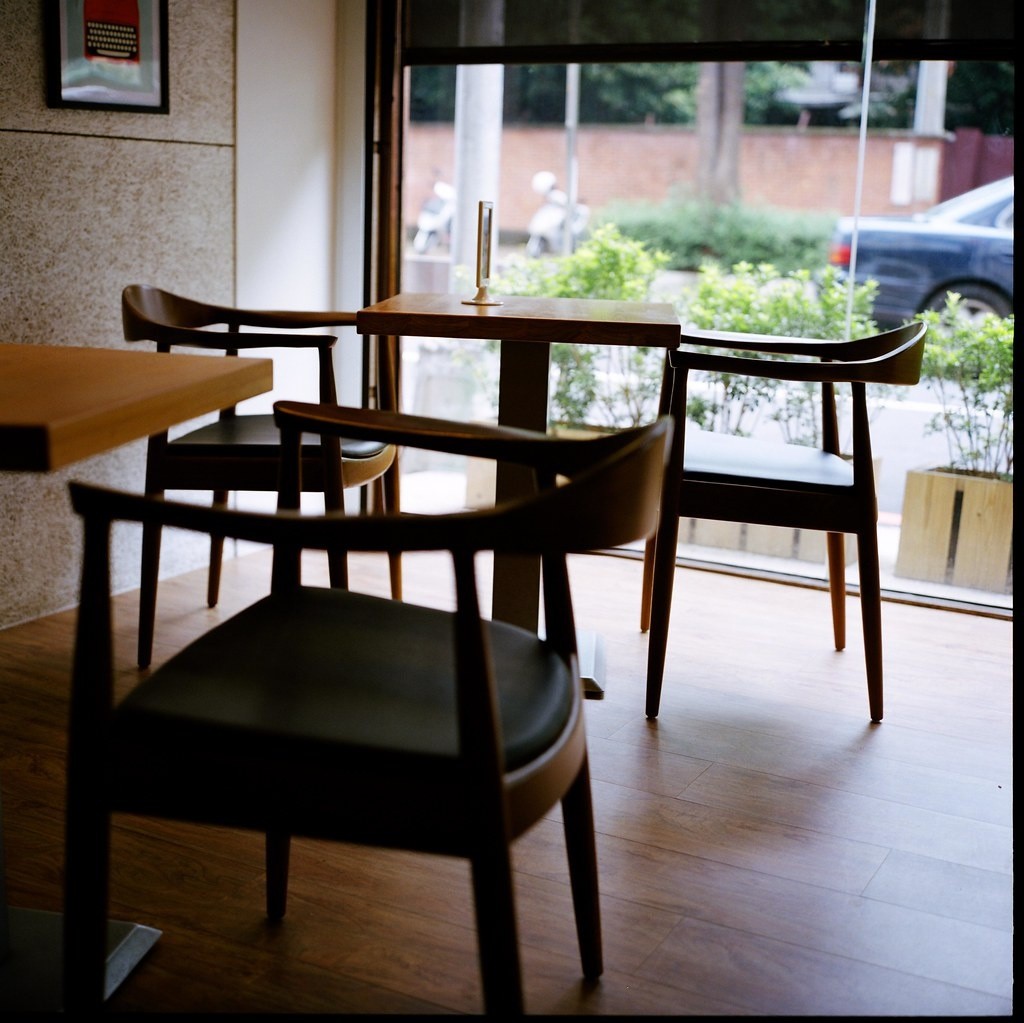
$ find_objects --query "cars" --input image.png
[811,176,1016,343]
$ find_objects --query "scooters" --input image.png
[412,182,456,254]
[525,169,590,261]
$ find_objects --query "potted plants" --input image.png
[896,292,1018,589]
[528,226,676,435]
[682,261,882,561]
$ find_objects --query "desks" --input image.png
[0,341,273,1023]
[353,293,680,702]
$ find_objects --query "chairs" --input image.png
[66,404,673,1023]
[637,320,926,721]
[118,282,399,668]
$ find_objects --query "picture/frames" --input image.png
[39,0,170,115]
